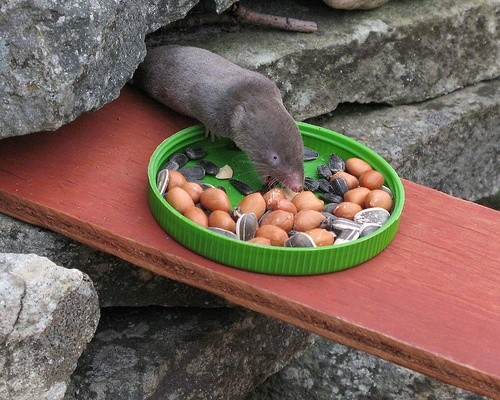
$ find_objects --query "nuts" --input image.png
[157,145,393,249]
